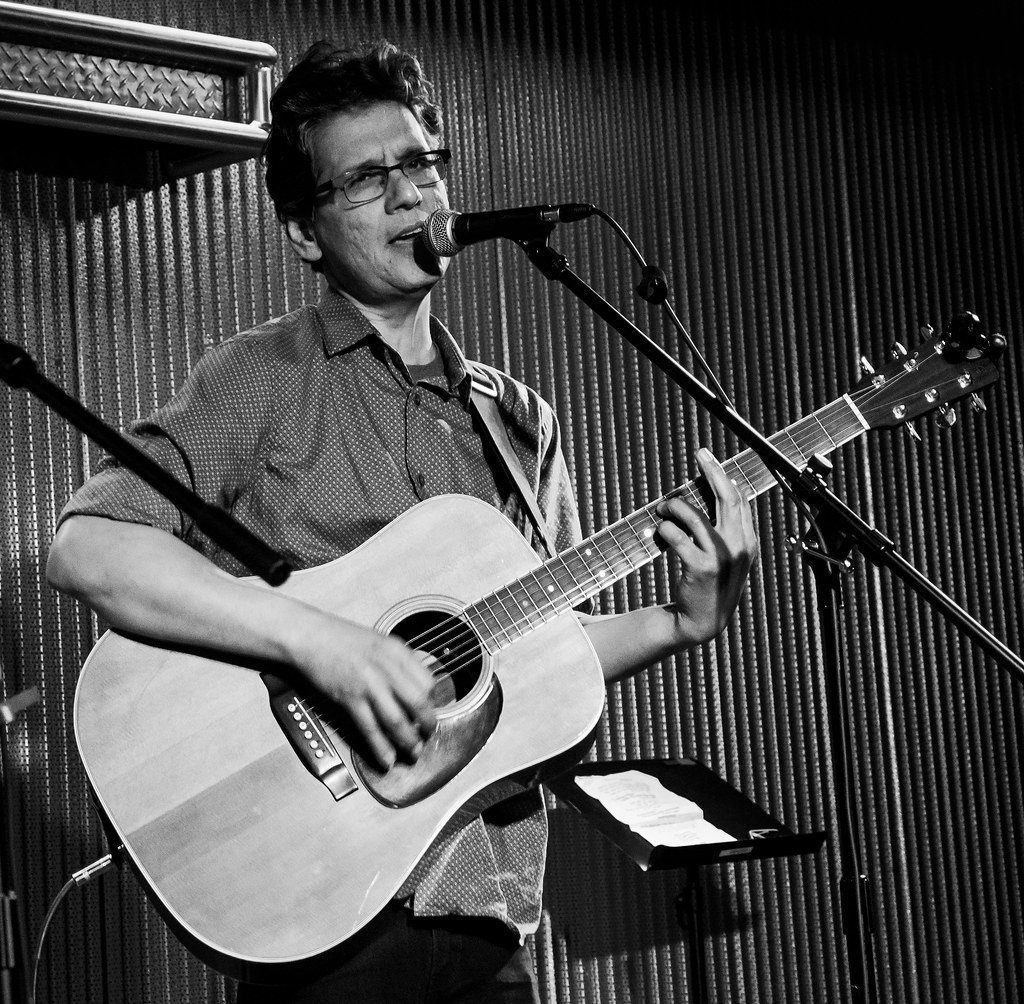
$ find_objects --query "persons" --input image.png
[46,43,757,1004]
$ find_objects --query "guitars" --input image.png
[67,305,1016,985]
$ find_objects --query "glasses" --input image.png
[312,149,451,205]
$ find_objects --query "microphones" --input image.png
[422,202,595,257]
